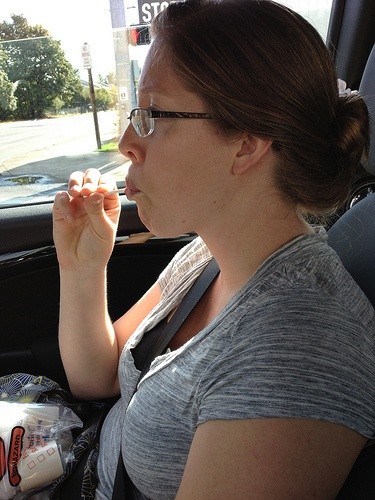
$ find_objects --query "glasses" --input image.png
[127,107,225,138]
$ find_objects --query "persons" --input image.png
[52,1,375,499]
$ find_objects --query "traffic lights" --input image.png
[131,26,150,45]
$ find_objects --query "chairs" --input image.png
[325,40,375,500]
